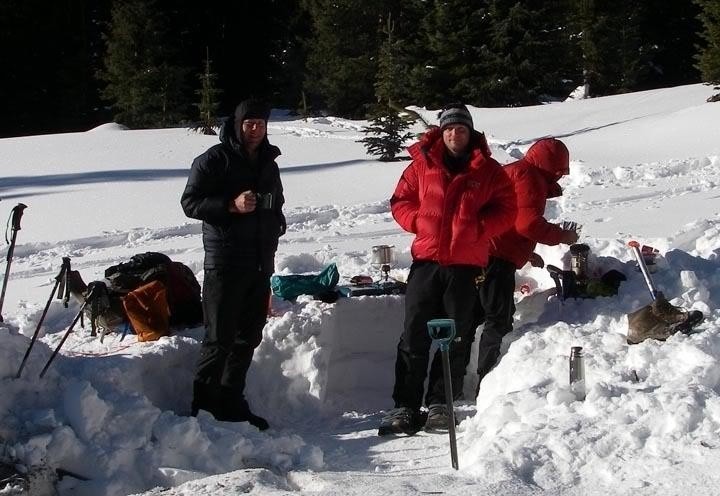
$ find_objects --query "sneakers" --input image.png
[378,404,458,435]
[192,405,268,429]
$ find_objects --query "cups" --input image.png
[563,221,577,244]
[255,191,273,209]
[569,346,586,401]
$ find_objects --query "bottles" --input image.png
[569,244,590,280]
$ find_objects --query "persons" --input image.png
[379,101,518,436]
[180,96,286,430]
[453,137,580,401]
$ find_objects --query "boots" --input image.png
[627,298,702,344]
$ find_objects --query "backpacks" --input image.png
[121,251,200,341]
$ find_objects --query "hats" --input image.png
[234,97,270,143]
[440,104,472,128]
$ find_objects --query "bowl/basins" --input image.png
[371,244,392,264]
